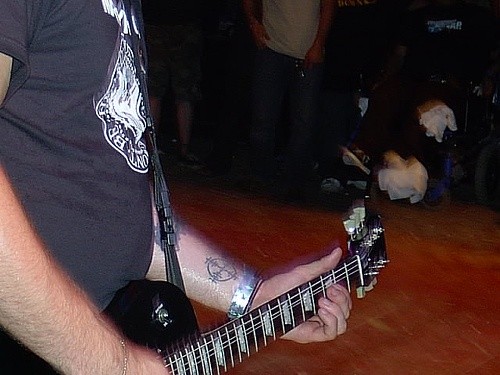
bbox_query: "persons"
[0,0,353,375]
[335,37,500,208]
[241,0,336,187]
[139,0,210,171]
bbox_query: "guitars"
[0,194,388,375]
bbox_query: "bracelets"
[226,268,265,321]
[118,334,129,375]
